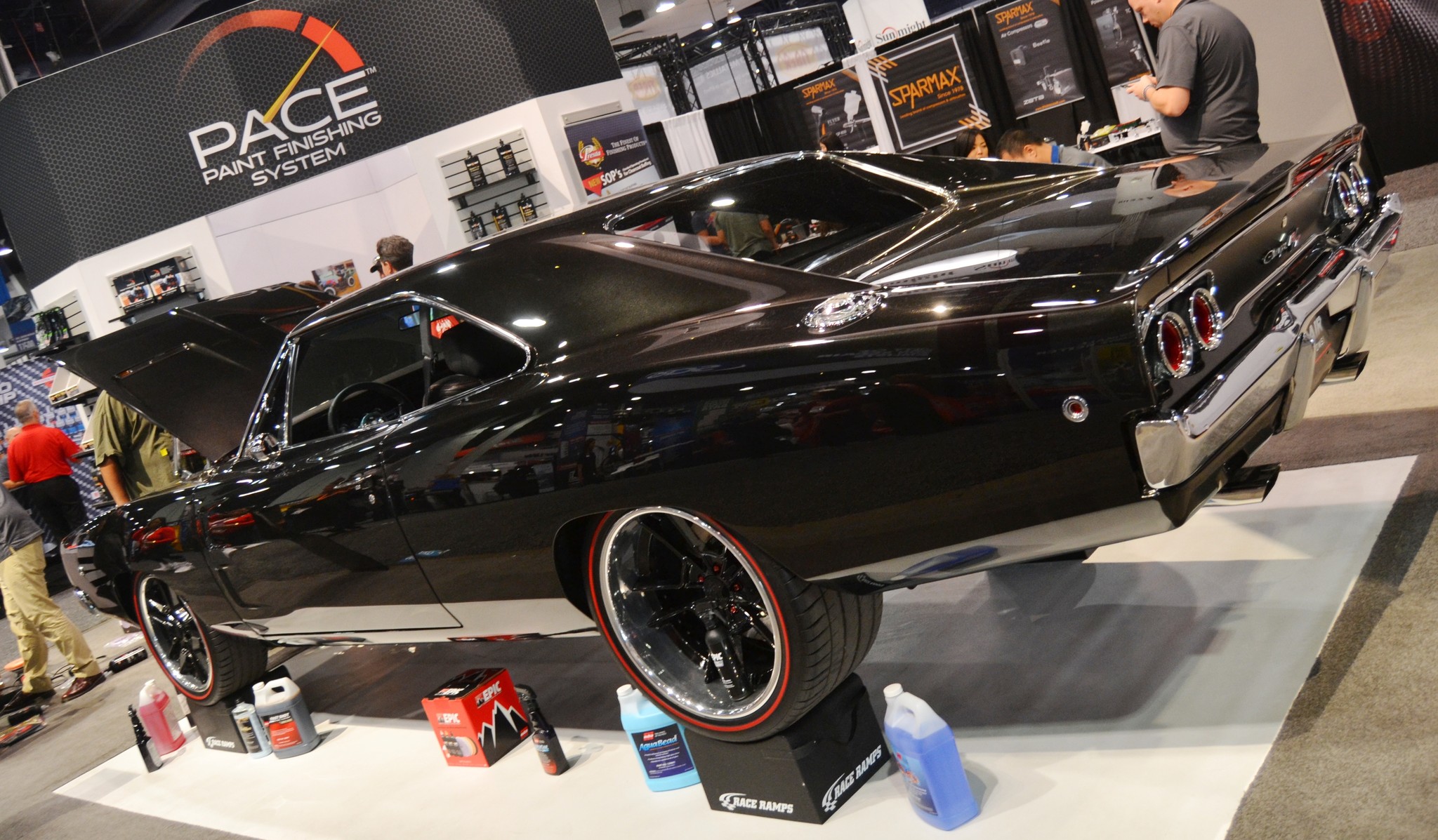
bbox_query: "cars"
[34,121,1402,745]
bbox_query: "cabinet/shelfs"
[435,126,550,245]
[107,284,210,327]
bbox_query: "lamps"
[725,0,743,25]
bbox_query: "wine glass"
[165,273,178,288]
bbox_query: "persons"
[369,259,384,281]
[691,210,730,256]
[0,425,24,490]
[1124,0,1261,157]
[90,389,179,506]
[953,127,990,159]
[130,517,176,561]
[577,437,599,485]
[377,236,414,276]
[0,483,107,713]
[93,509,129,579]
[716,212,779,261]
[8,399,89,543]
[819,132,843,151]
[995,127,1112,168]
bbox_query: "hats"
[369,255,386,273]
[375,234,415,264]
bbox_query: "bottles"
[175,685,195,728]
[88,465,114,502]
[492,202,512,232]
[517,193,538,223]
[8,704,50,727]
[617,684,700,790]
[883,682,980,830]
[524,693,570,775]
[232,698,273,758]
[441,730,463,757]
[129,711,163,772]
[252,677,321,759]
[134,285,146,300]
[463,150,488,189]
[467,211,488,241]
[106,646,148,673]
[496,138,520,178]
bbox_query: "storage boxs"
[186,665,304,755]
[685,671,890,827]
[420,666,535,770]
[112,254,197,316]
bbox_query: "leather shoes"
[61,672,108,703]
[3,685,57,714]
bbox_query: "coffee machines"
[139,679,184,756]
